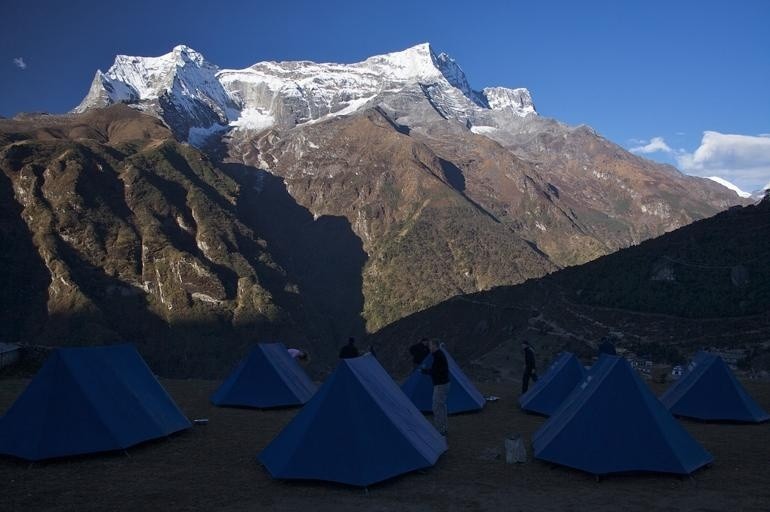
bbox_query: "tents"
[516,351,585,417]
[531,354,720,479]
[398,343,488,416]
[1,339,197,468]
[255,352,450,487]
[518,340,538,394]
[207,339,317,408]
[654,352,769,424]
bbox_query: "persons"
[410,337,428,367]
[337,335,358,359]
[287,344,308,361]
[421,339,451,437]
[370,346,376,356]
